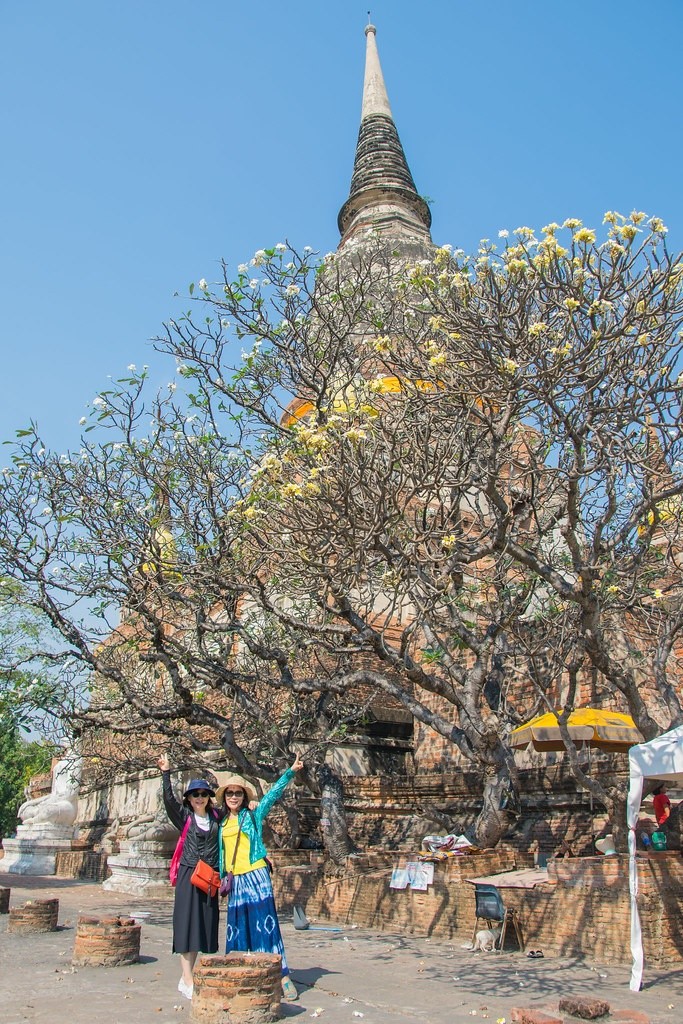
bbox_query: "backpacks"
[169,810,218,887]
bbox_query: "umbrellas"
[506,706,645,856]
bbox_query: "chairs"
[469,884,524,955]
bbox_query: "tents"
[627,725,683,991]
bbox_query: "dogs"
[470,925,502,952]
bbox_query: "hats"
[183,780,216,797]
[215,776,254,806]
[594,834,615,853]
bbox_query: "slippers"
[527,950,537,958]
[536,951,543,957]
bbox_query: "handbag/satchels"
[219,871,233,897]
[190,859,221,906]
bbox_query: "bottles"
[652,831,667,851]
[641,831,653,850]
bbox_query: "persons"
[17,737,83,824]
[120,770,186,841]
[157,752,259,999]
[652,784,671,828]
[215,752,304,1000]
[101,811,120,841]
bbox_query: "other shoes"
[282,978,297,1000]
[178,979,194,999]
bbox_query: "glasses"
[189,791,209,797]
[225,791,244,797]
